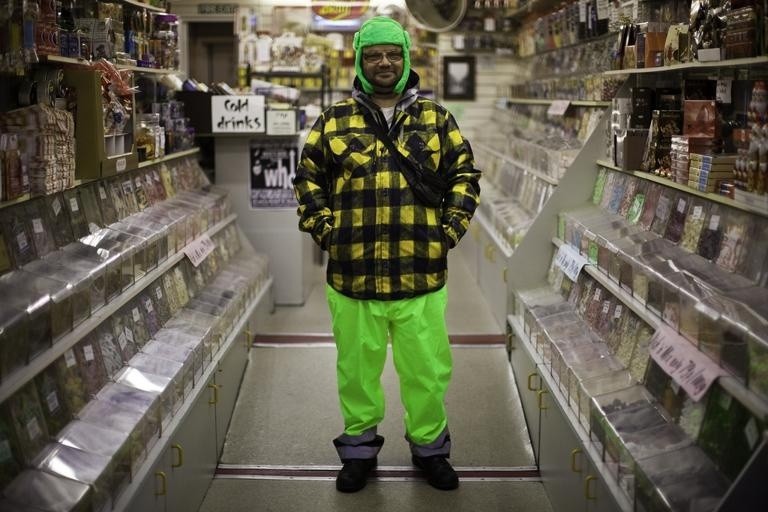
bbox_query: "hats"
[352,16,413,96]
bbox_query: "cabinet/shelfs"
[1,0,277,510]
[213,103,322,307]
[453,0,768,512]
[243,32,337,113]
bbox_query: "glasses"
[361,52,403,64]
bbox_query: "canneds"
[136,114,160,160]
[150,113,165,160]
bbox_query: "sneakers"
[412,453,460,491]
[335,454,377,494]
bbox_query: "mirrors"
[404,2,469,32]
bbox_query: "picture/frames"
[440,55,476,100]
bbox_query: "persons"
[291,17,484,494]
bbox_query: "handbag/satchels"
[395,152,448,207]
